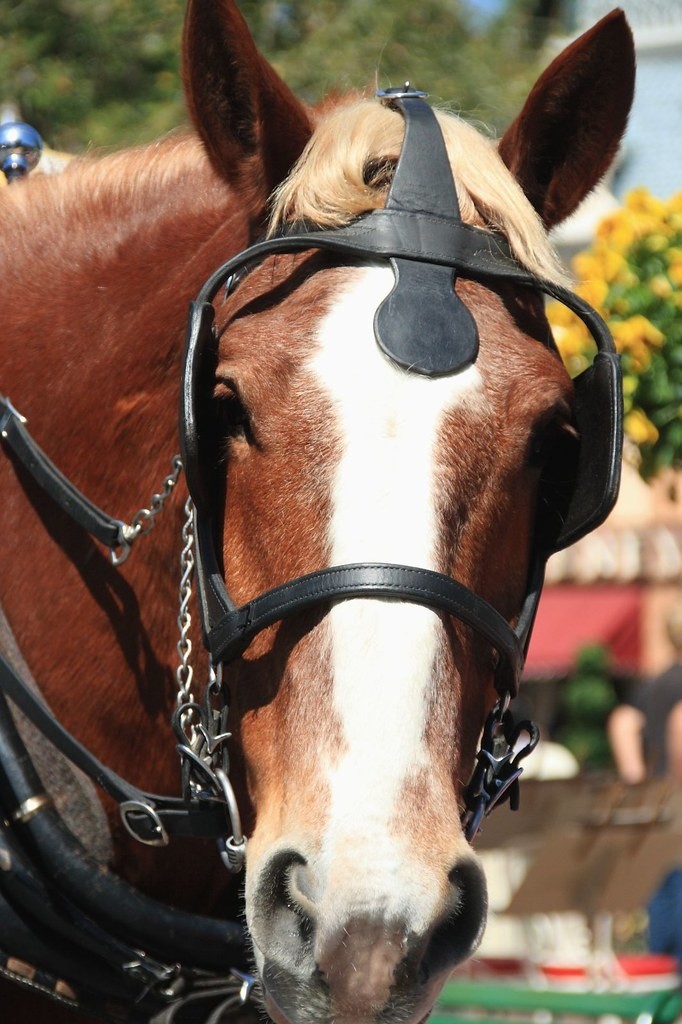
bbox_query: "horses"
[1,0,640,1024]
